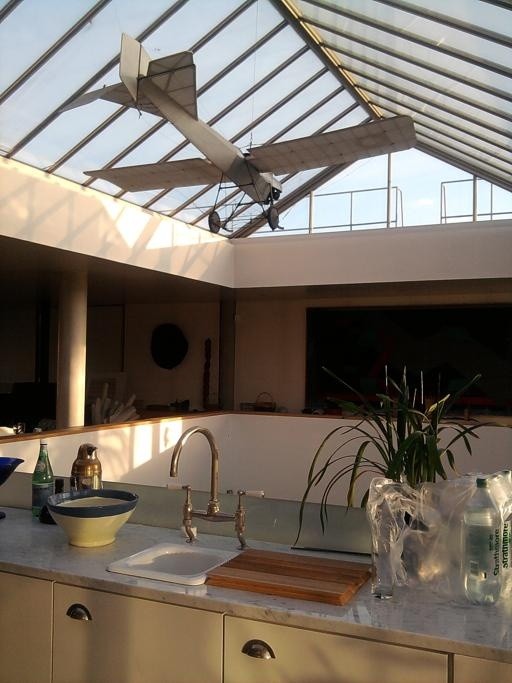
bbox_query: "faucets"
[170,425,221,513]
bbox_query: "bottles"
[31,439,54,517]
[457,470,512,604]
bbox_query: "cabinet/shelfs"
[452,653,512,683]
[223,615,450,683]
[0,571,54,683]
[51,582,224,683]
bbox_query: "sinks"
[108,541,236,586]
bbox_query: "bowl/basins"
[46,488,139,547]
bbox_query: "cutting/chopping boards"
[205,549,377,605]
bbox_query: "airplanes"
[55,32,421,235]
